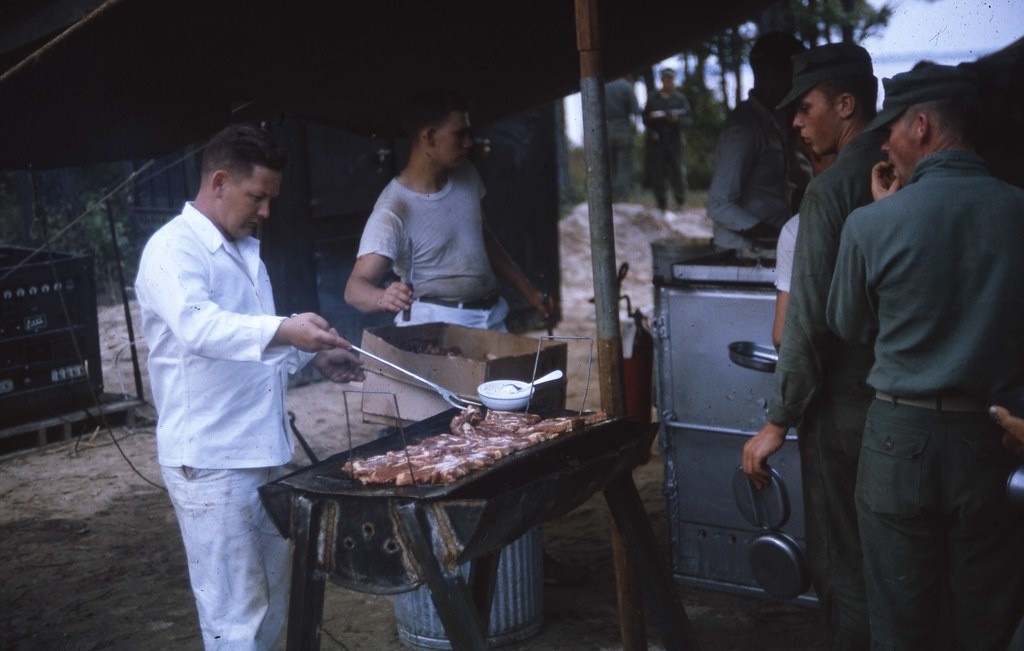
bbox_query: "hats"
[662,69,676,77]
[776,44,872,110]
[862,61,976,134]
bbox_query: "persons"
[344,91,556,332]
[826,59,1024,651]
[706,31,817,268]
[743,41,902,651]
[643,69,694,211]
[134,124,365,651]
[605,77,643,204]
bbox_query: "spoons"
[499,370,563,393]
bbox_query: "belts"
[418,294,500,309]
[874,386,995,414]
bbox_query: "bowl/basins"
[478,380,535,410]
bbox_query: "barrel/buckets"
[394,523,544,651]
[649,239,716,319]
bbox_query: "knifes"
[402,238,413,321]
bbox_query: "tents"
[0,0,768,651]
